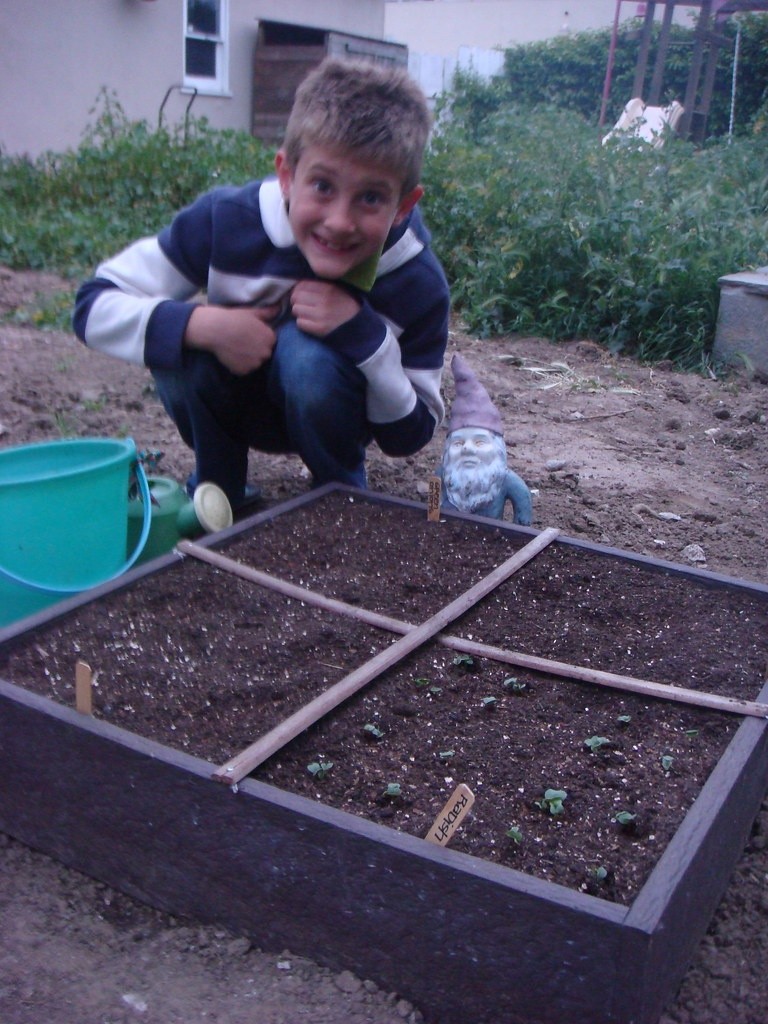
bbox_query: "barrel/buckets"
[0,437,152,629]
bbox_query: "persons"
[438,352,535,526]
[71,54,451,520]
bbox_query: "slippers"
[181,483,260,510]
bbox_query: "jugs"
[124,474,233,566]
